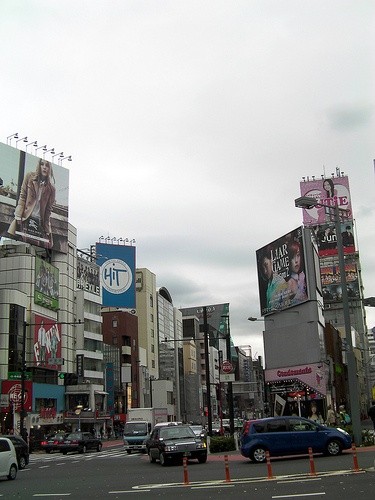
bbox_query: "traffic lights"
[23,428,28,438]
[60,372,77,380]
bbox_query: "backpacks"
[345,413,351,424]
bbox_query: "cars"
[0,438,18,480]
[1,435,29,469]
[41,434,67,453]
[61,431,103,455]
[240,416,353,463]
[155,419,246,440]
[145,425,207,466]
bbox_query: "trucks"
[122,407,168,454]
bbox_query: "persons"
[326,405,336,428]
[36,261,55,298]
[38,320,47,366]
[100,425,111,440]
[282,241,307,303]
[78,262,100,296]
[339,405,350,432]
[7,160,55,248]
[49,328,58,368]
[329,226,337,248]
[341,226,354,247]
[320,227,330,249]
[50,424,72,437]
[75,427,96,439]
[113,425,124,440]
[8,428,16,436]
[22,427,44,455]
[256,246,287,313]
[369,401,375,430]
[308,402,321,424]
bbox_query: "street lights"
[295,196,364,444]
[221,314,235,438]
